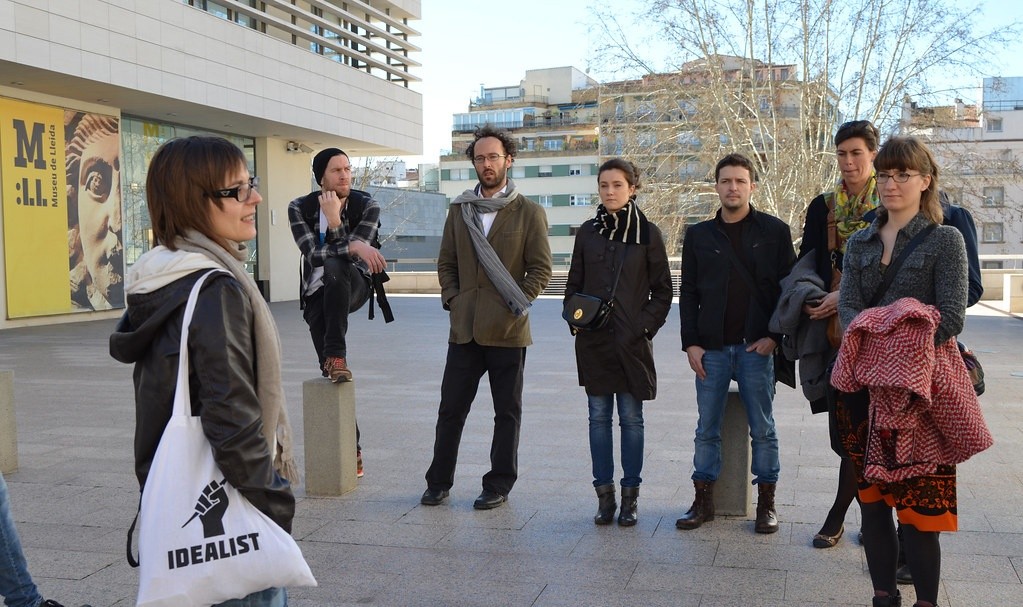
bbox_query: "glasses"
[872,170,920,184]
[840,121,876,137]
[204,176,259,203]
[472,155,506,163]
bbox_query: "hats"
[312,148,347,185]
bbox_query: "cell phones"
[805,299,823,308]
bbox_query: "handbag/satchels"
[136,268,317,607]
[961,350,983,396]
[564,291,611,331]
[828,268,845,348]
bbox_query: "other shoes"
[814,524,844,548]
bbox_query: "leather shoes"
[895,562,914,584]
[473,488,509,508]
[421,489,450,506]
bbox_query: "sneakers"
[355,452,364,476]
[325,356,353,383]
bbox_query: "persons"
[0,472,45,607]
[675,154,798,532]
[110,136,296,607]
[562,158,674,525]
[66,113,126,310]
[837,134,984,607]
[420,124,552,510]
[799,120,881,550]
[288,148,388,476]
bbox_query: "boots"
[593,483,616,524]
[619,486,642,523]
[676,479,715,529]
[756,481,780,533]
